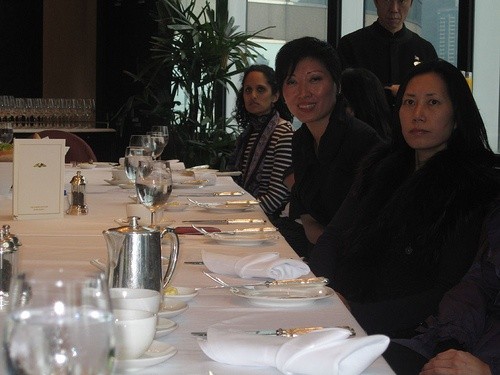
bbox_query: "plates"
[104,179,127,185]
[161,202,190,211]
[173,179,209,189]
[118,184,130,189]
[203,205,247,213]
[76,162,118,167]
[155,287,199,337]
[114,219,131,226]
[205,235,279,245]
[113,340,177,371]
[229,287,334,307]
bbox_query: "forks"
[192,224,279,236]
[203,271,331,289]
[187,198,261,208]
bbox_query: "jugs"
[90,216,179,294]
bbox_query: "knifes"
[179,192,244,196]
[192,326,355,337]
[182,218,266,224]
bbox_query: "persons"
[274,36,500,375]
[233,65,296,220]
[336,0,437,98]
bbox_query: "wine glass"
[125,125,173,228]
[0,95,97,128]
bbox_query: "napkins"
[198,323,390,374]
[202,252,310,280]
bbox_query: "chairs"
[30,130,97,163]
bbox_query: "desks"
[12,128,116,161]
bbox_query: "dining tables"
[0,159,395,375]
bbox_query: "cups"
[461,70,472,93]
[0,122,14,144]
[113,309,157,360]
[112,169,124,179]
[109,288,162,314]
[4,266,112,375]
[126,203,155,225]
[119,158,125,166]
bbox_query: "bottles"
[0,224,21,291]
[70,172,88,214]
